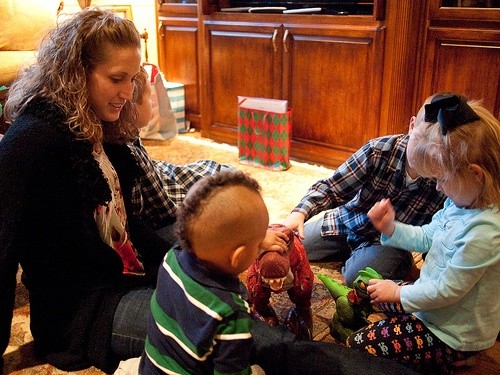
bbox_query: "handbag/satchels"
[136,61,178,142]
[236,95,294,172]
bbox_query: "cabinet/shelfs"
[155,3,201,132]
[417,8,500,121]
[198,12,382,164]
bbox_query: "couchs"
[0,0,83,102]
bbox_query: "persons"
[0,8,395,375]
[278,88,448,289]
[345,97,499,375]
[137,168,290,375]
